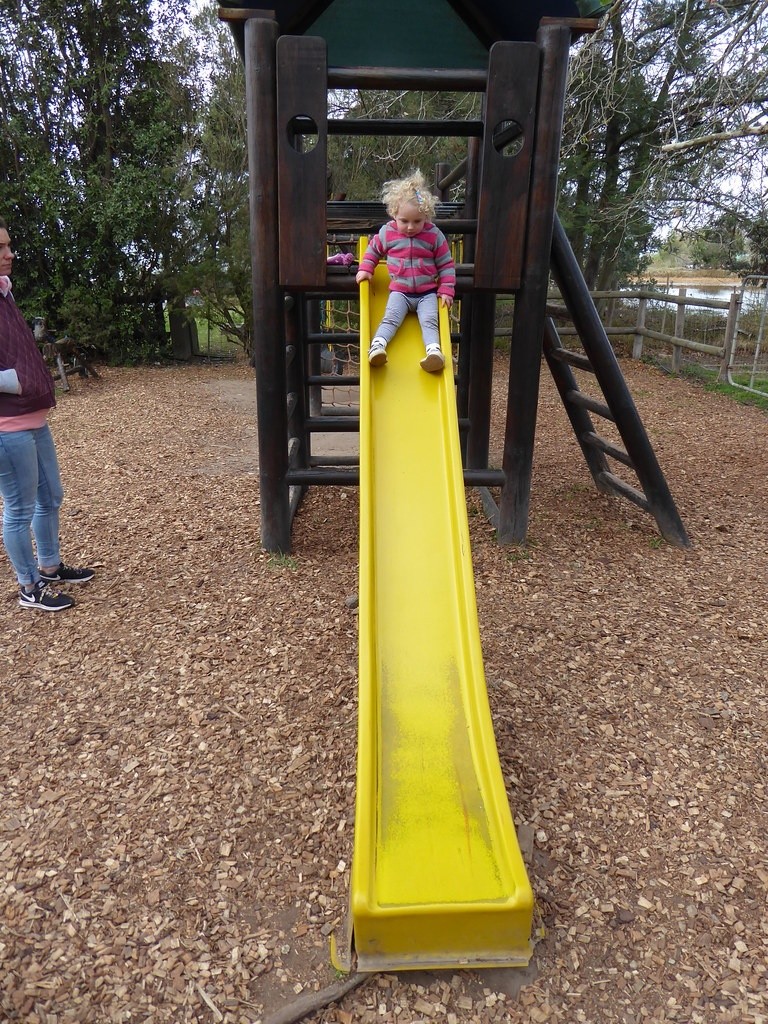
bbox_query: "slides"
[345,234,539,975]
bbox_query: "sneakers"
[37,562,95,584]
[19,584,75,612]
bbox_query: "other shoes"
[419,349,445,373]
[368,342,388,368]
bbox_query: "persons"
[354,169,460,374]
[0,212,95,611]
